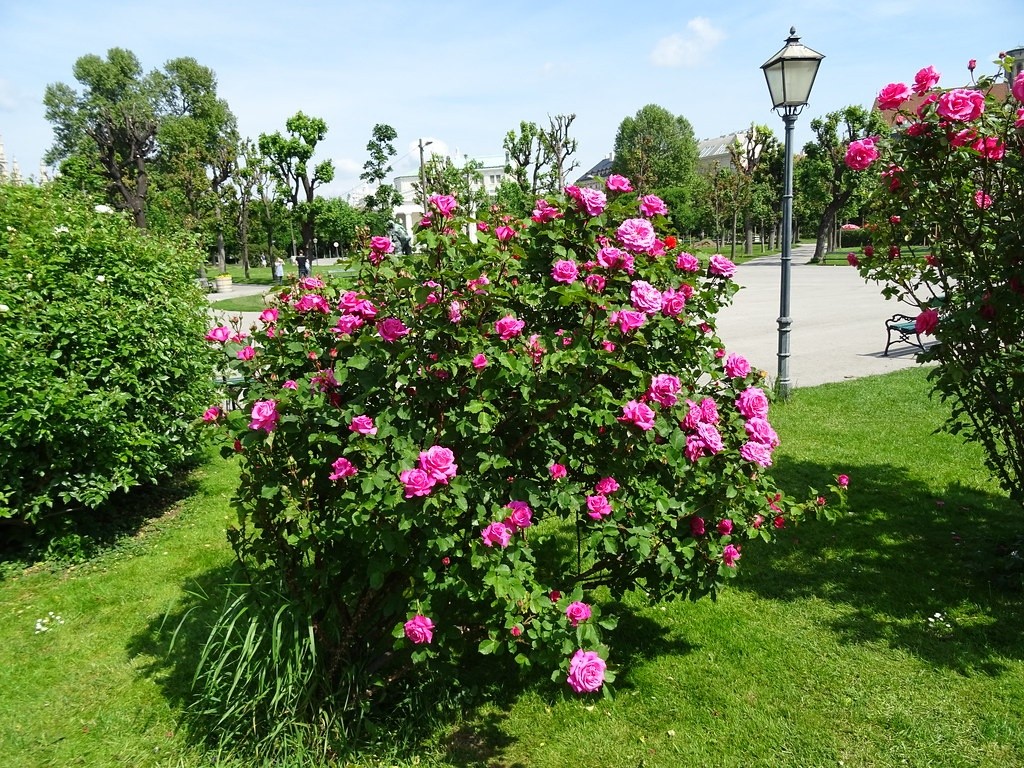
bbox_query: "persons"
[261,252,266,268]
[274,257,285,286]
[295,250,309,282]
[387,220,413,256]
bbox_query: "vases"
[216,279,232,292]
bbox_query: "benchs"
[883,314,928,356]
[195,278,214,293]
[328,268,357,281]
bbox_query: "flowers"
[214,272,232,279]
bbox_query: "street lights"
[759,26,827,401]
[313,237,320,268]
[419,139,435,217]
